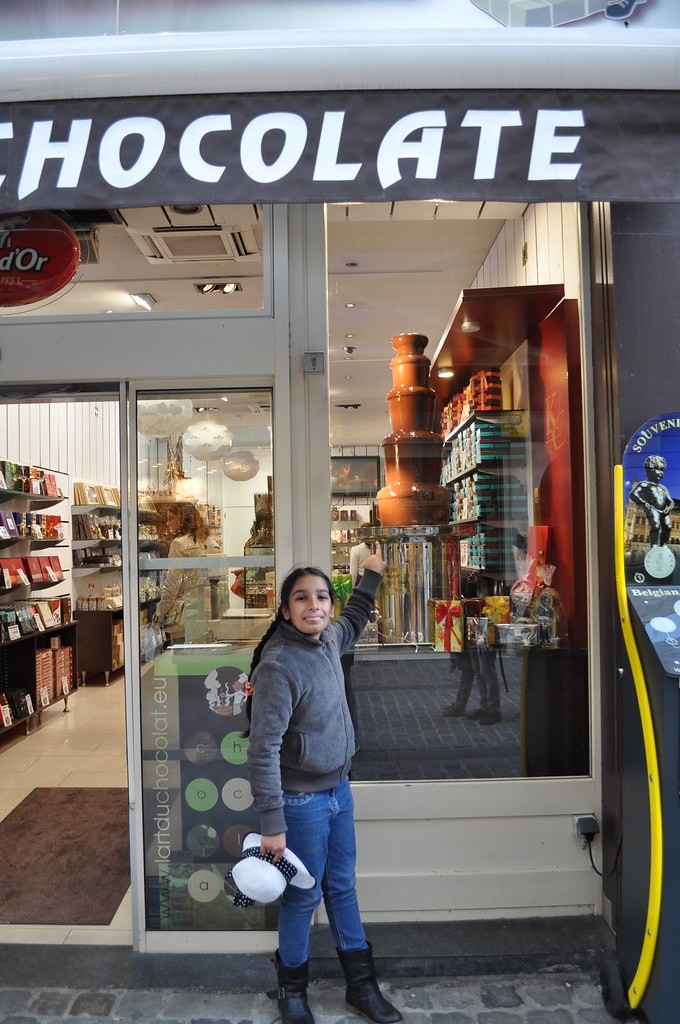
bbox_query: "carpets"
[0,787,181,926]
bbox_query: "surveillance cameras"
[344,347,356,354]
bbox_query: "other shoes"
[441,703,466,717]
[478,711,501,725]
[468,706,487,719]
[448,663,456,672]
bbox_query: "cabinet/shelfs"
[71,502,158,579]
[72,596,171,686]
[0,457,79,736]
[442,408,526,580]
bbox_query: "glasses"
[166,516,176,524]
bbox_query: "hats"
[225,833,316,908]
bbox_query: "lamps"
[127,399,193,438]
[460,321,480,332]
[222,449,259,481]
[221,283,236,295]
[199,284,216,295]
[437,368,456,378]
[182,399,232,461]
[130,294,154,311]
[196,408,204,412]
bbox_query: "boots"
[336,939,404,1024]
[270,945,315,1024]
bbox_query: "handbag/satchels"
[142,626,167,662]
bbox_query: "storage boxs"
[427,368,526,653]
[1,462,73,727]
[112,609,149,665]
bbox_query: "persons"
[444,545,501,725]
[244,506,275,611]
[346,523,377,623]
[152,503,222,645]
[245,539,404,1024]
